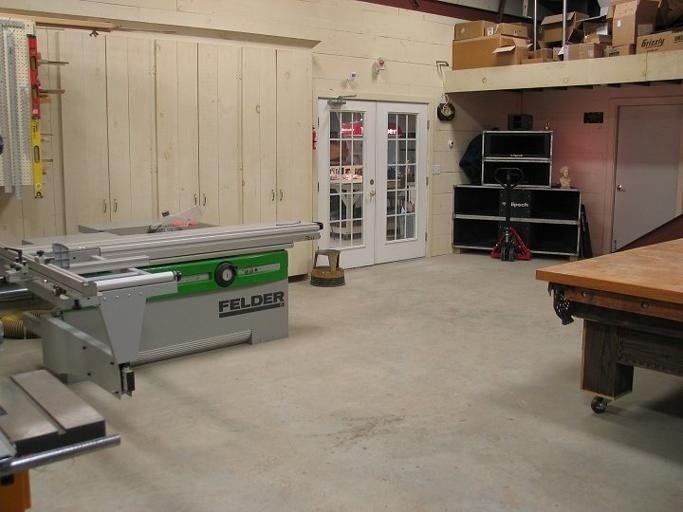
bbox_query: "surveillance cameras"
[346,71,357,81]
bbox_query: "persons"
[558,166,572,189]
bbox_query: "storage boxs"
[452,1,683,69]
